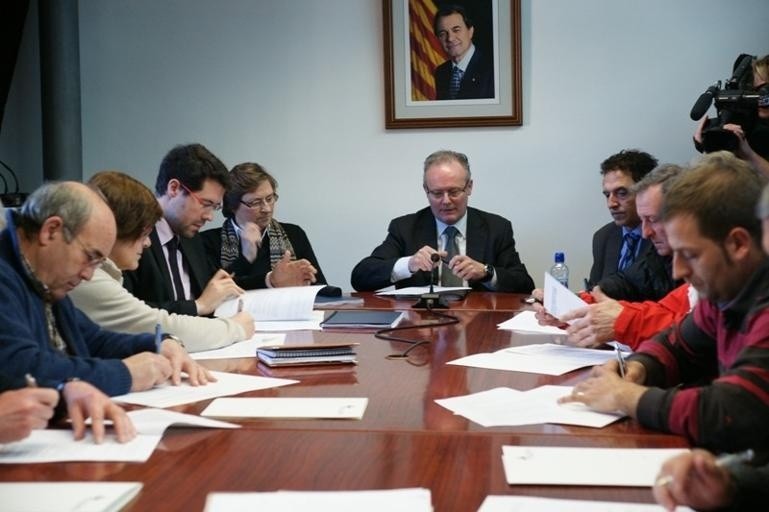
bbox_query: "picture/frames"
[380,0,524,133]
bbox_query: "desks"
[0,287,720,511]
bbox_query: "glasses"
[65,223,105,271]
[240,193,279,208]
[426,180,469,197]
[180,183,222,211]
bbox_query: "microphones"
[411,254,450,308]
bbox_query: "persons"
[351,151,536,292]
[531,150,769,352]
[430,4,494,99]
[690,52,768,165]
[555,147,769,446]
[198,162,329,286]
[0,180,218,445]
[652,183,769,511]
[66,170,255,352]
[122,142,317,318]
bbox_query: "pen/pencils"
[24,373,37,387]
[614,342,626,377]
[441,257,466,277]
[657,448,755,488]
[238,299,243,312]
[155,324,161,353]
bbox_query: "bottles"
[550,252,570,289]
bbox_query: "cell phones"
[520,295,535,303]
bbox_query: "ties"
[618,233,638,270]
[165,235,186,303]
[440,226,464,288]
[448,66,461,99]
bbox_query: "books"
[319,311,404,328]
[256,343,358,368]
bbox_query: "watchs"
[479,264,494,282]
